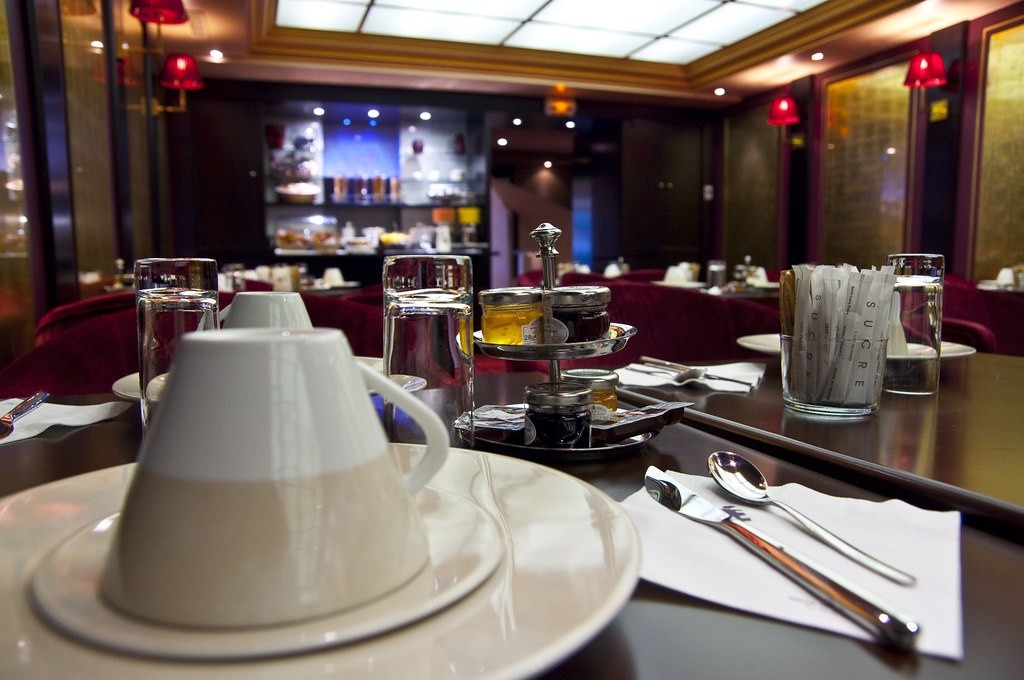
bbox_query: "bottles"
[562,368,618,415]
[552,286,610,344]
[479,287,544,346]
[734,265,748,293]
[525,383,592,449]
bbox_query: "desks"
[2,392,1023,678]
[614,353,1024,522]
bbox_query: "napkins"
[0,397,131,444]
[617,363,767,391]
[611,468,964,658]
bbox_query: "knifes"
[0,391,51,440]
[644,464,919,649]
[641,355,751,386]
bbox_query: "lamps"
[905,52,959,112]
[767,89,808,149]
[124,1,189,56]
[153,52,203,114]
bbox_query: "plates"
[1,441,639,675]
[34,489,505,660]
[111,356,386,400]
[737,333,977,360]
[652,281,705,289]
[755,283,781,288]
[146,373,427,402]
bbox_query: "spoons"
[627,364,705,383]
[709,451,915,586]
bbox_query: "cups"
[324,171,401,201]
[778,335,891,421]
[882,253,945,395]
[222,264,301,293]
[136,259,219,438]
[97,288,444,630]
[382,255,475,450]
[707,260,728,289]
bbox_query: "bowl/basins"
[275,185,320,202]
